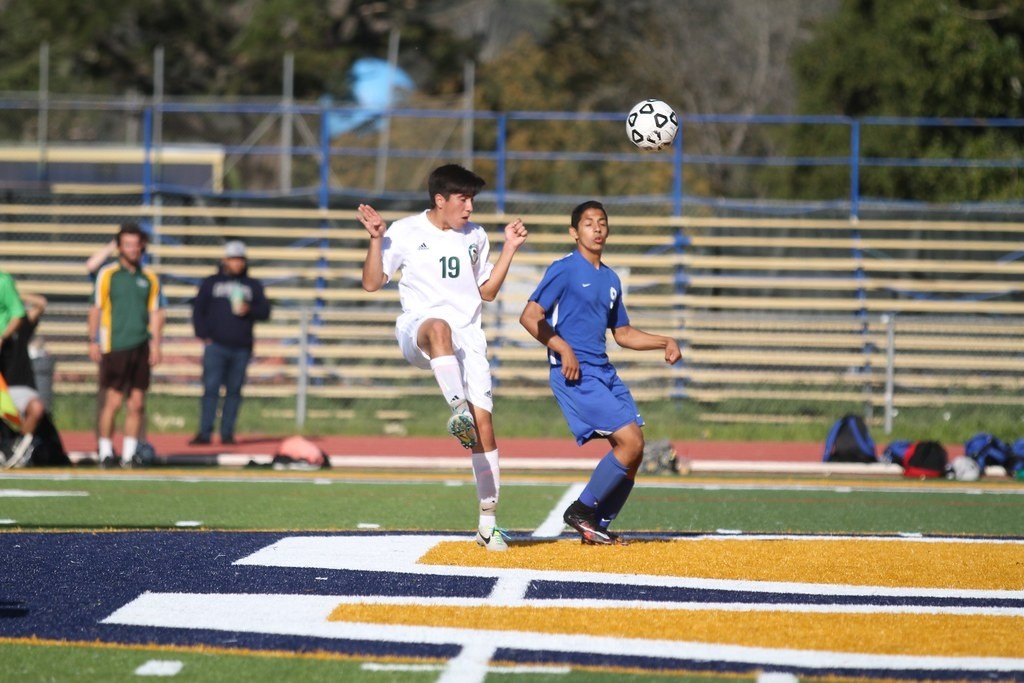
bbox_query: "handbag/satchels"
[886,435,1024,481]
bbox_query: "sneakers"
[476,526,511,551]
[581,534,627,545]
[563,503,612,544]
[449,412,478,449]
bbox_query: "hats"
[224,242,245,258]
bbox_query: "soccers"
[952,455,980,482]
[626,99,679,152]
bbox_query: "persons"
[188,241,271,446]
[520,201,681,546]
[0,272,71,466]
[85,223,167,469]
[355,163,528,550]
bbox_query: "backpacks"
[824,412,876,461]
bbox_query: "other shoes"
[224,439,236,445]
[190,436,210,446]
